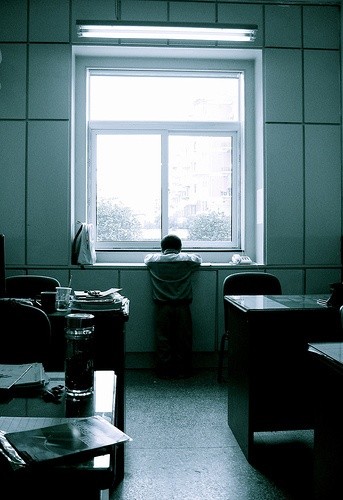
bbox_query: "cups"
[56,287,72,311]
[35,292,57,312]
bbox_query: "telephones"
[231,253,253,265]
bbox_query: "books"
[0,363,49,389]
[0,415,129,473]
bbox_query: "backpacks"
[72,220,97,265]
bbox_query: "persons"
[146,236,200,379]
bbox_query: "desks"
[0,297,130,500]
[223,293,343,490]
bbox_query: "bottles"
[65,314,95,396]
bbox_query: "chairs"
[5,276,61,300]
[216,273,282,383]
[1,299,52,367]
